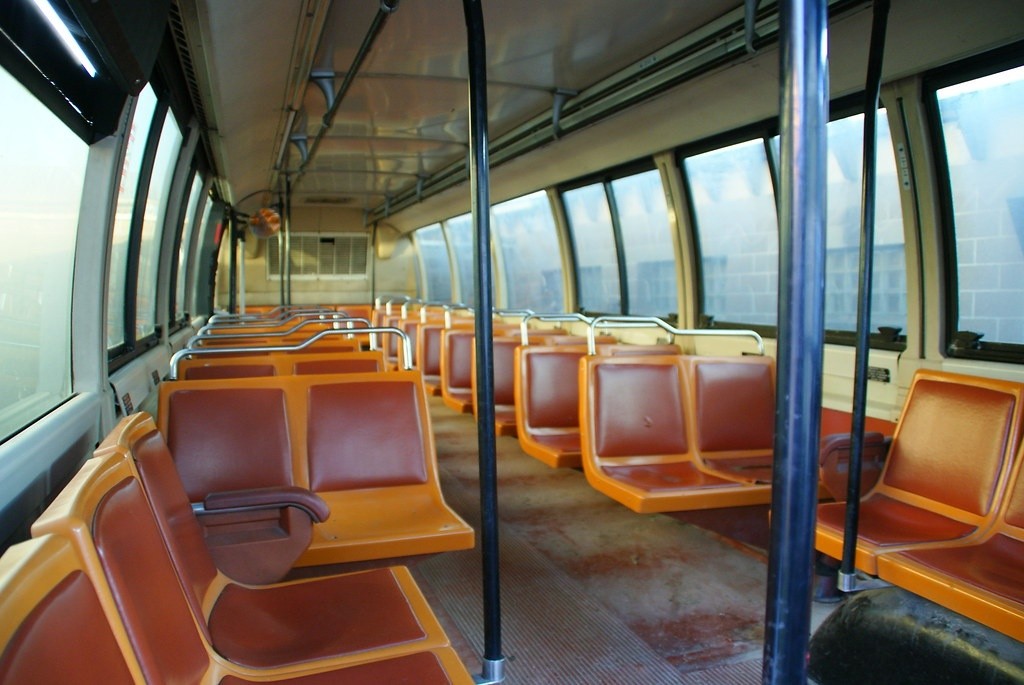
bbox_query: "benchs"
[0,293,1024,685]
